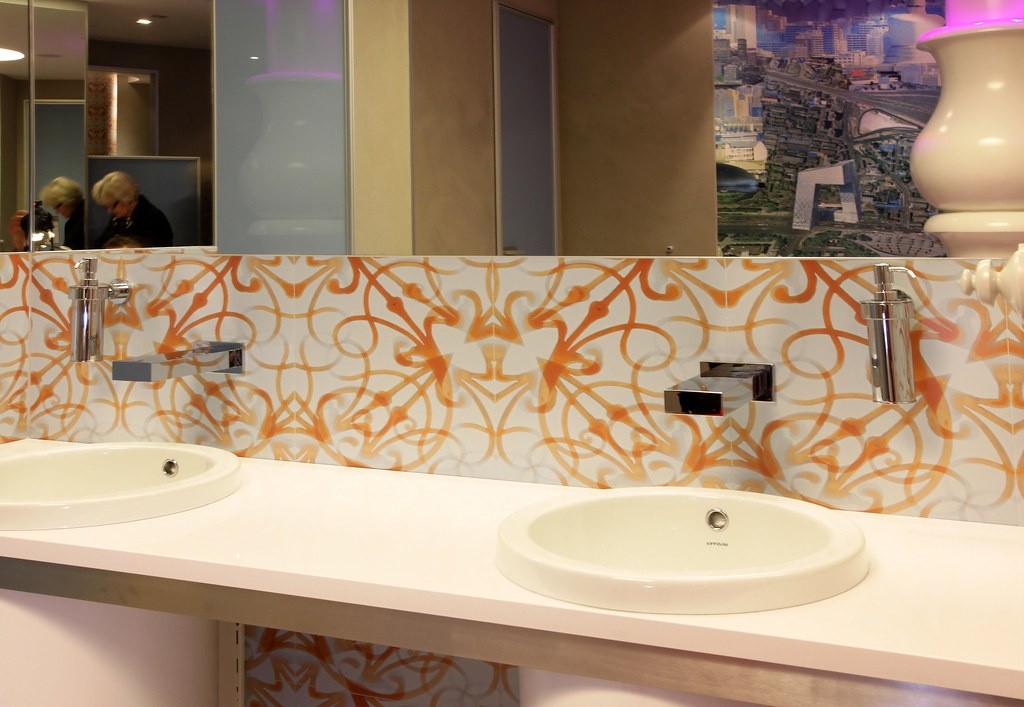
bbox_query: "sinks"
[0,442,241,530]
[497,487,870,615]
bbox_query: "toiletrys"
[860,262,919,403]
[67,256,109,364]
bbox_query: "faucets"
[111,341,246,382]
[663,361,775,416]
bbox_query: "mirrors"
[0,0,1024,258]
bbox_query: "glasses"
[54,204,62,210]
[105,200,119,210]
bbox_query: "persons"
[93,169,175,247]
[38,177,85,250]
